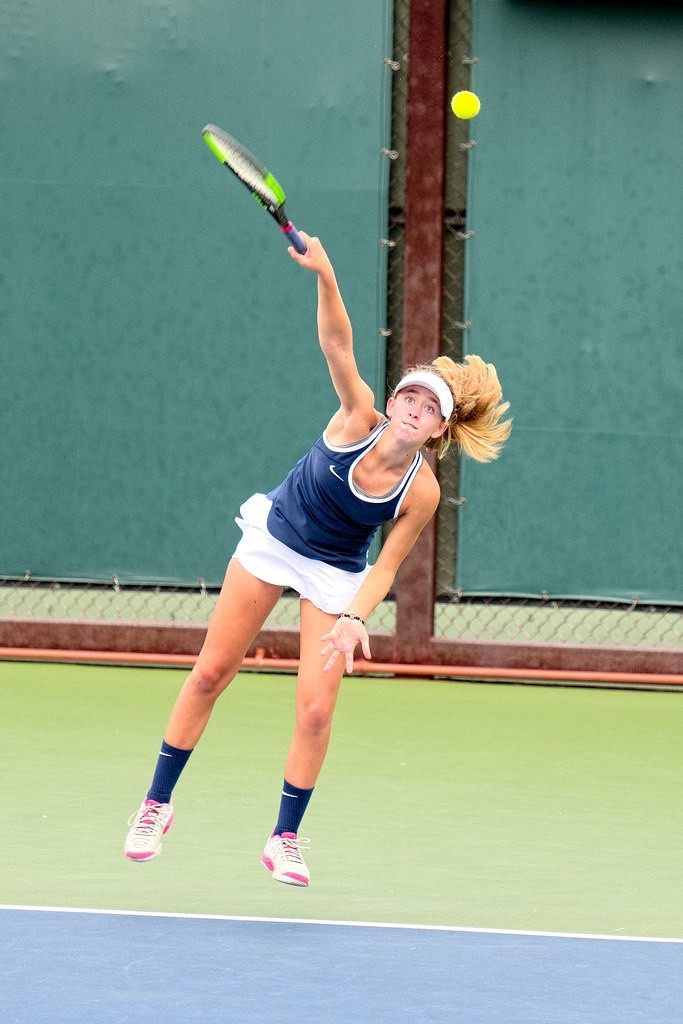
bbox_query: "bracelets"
[337,614,365,625]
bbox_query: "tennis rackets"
[201,124,307,255]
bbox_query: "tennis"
[450,89,482,121]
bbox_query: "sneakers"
[259,828,309,887]
[124,796,176,862]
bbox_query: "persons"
[123,228,514,888]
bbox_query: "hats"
[393,371,453,422]
